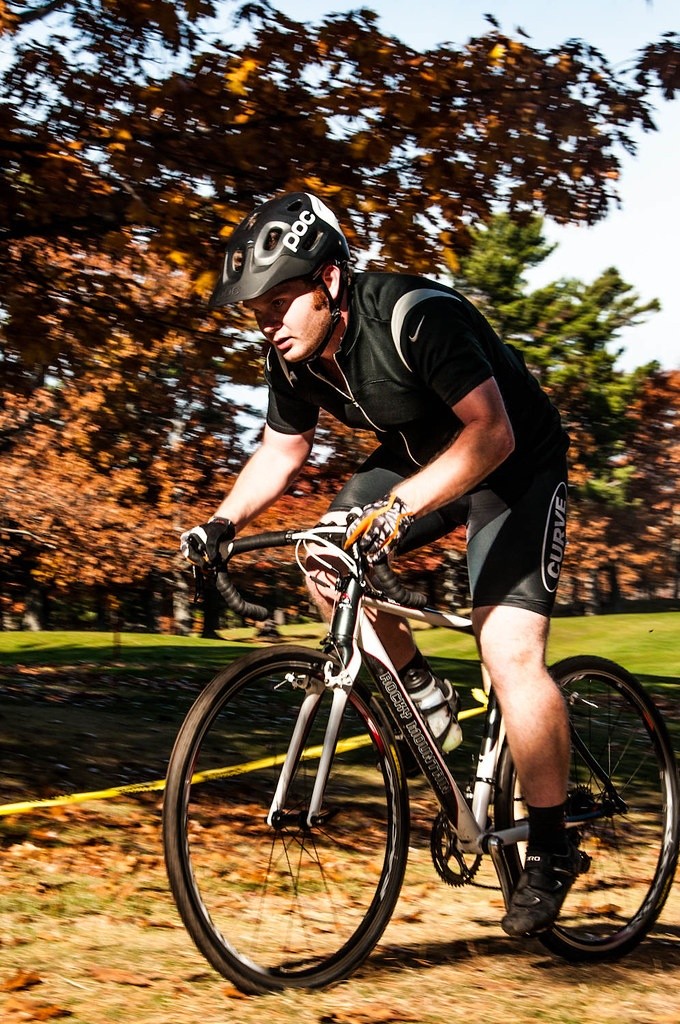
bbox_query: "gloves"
[180,515,235,574]
[344,491,416,565]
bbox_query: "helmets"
[208,192,351,306]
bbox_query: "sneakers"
[376,674,457,778]
[500,827,582,940]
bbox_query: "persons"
[178,191,592,936]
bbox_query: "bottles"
[403,668,463,754]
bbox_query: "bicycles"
[159,505,680,996]
